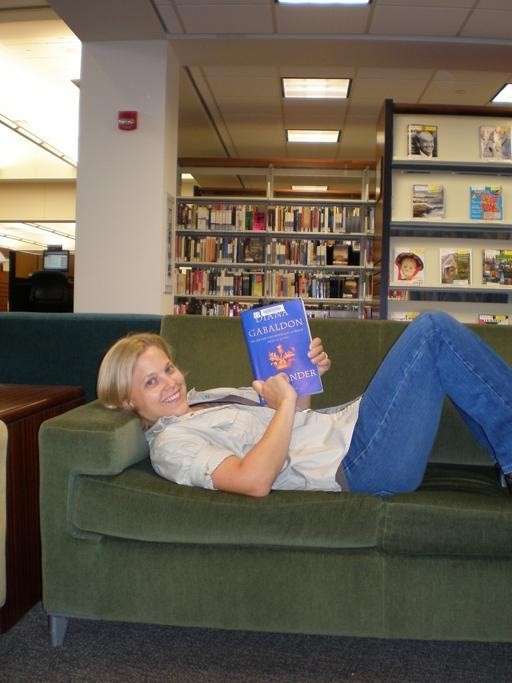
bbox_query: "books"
[407,123,440,161]
[479,126,511,161]
[175,201,373,318]
[481,248,512,289]
[478,313,510,325]
[470,183,504,223]
[410,183,446,221]
[439,248,472,288]
[392,246,426,286]
[240,296,324,403]
[387,310,420,321]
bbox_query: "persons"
[97,309,511,499]
[413,131,437,156]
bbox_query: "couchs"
[37,317,511,647]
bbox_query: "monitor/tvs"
[42,250,69,273]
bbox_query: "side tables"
[0,383,88,633]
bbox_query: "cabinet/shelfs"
[173,194,383,319]
[371,99,512,320]
